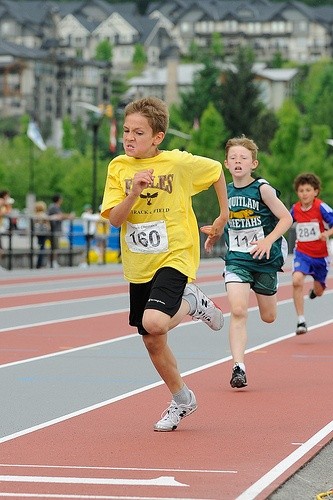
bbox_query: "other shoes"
[309,288,317,300]
[296,321,307,335]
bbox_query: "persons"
[49,194,76,268]
[205,137,293,389]
[34,200,68,269]
[99,97,229,432]
[0,190,15,231]
[288,173,333,334]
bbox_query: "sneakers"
[153,390,198,431]
[230,361,246,389]
[182,282,223,331]
